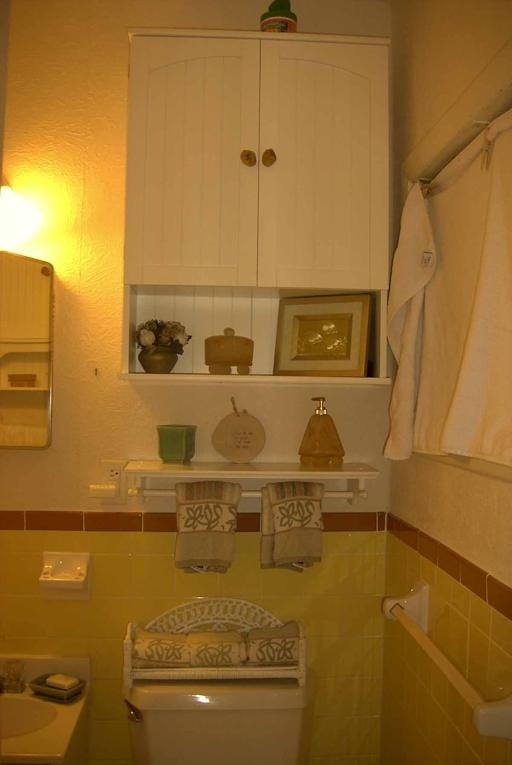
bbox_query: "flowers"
[133,319,191,355]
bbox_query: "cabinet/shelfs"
[114,26,394,387]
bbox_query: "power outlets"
[97,458,127,506]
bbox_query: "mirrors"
[0,251,55,451]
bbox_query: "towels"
[382,126,512,467]
[259,481,325,574]
[132,621,300,669]
[173,480,243,576]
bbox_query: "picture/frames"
[272,293,373,378]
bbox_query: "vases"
[137,345,177,373]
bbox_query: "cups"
[154,420,199,466]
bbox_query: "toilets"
[125,679,307,765]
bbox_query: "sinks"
[0,684,90,764]
